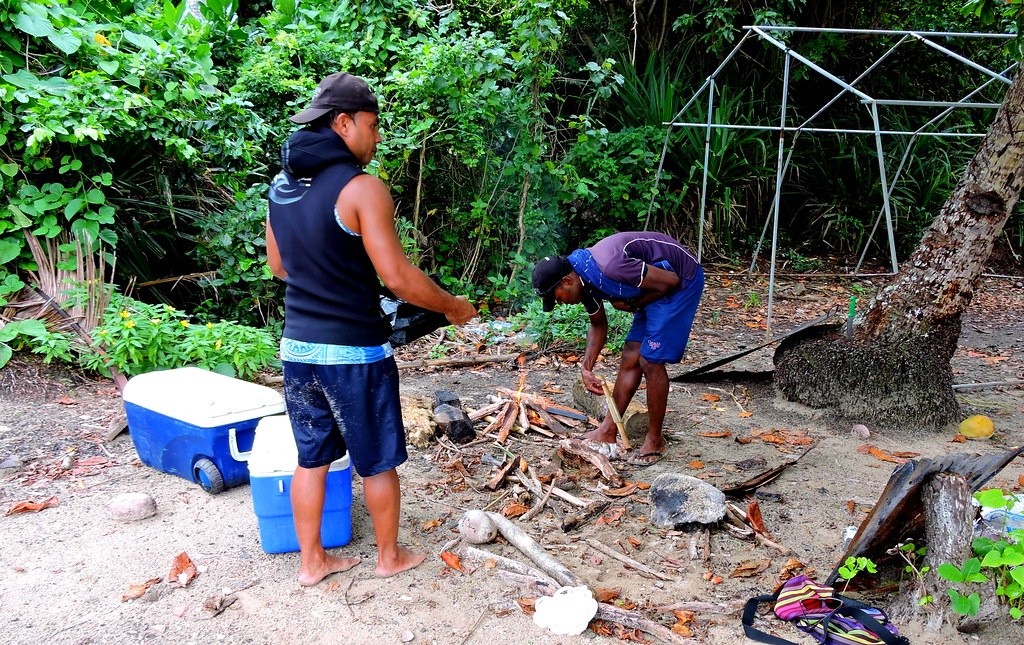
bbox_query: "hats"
[288,72,379,124]
[532,256,571,312]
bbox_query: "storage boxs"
[122,366,288,496]
[246,413,353,554]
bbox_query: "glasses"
[799,598,844,629]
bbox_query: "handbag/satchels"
[742,576,910,645]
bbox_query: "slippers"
[630,446,669,466]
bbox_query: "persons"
[532,231,704,465]
[267,72,478,586]
